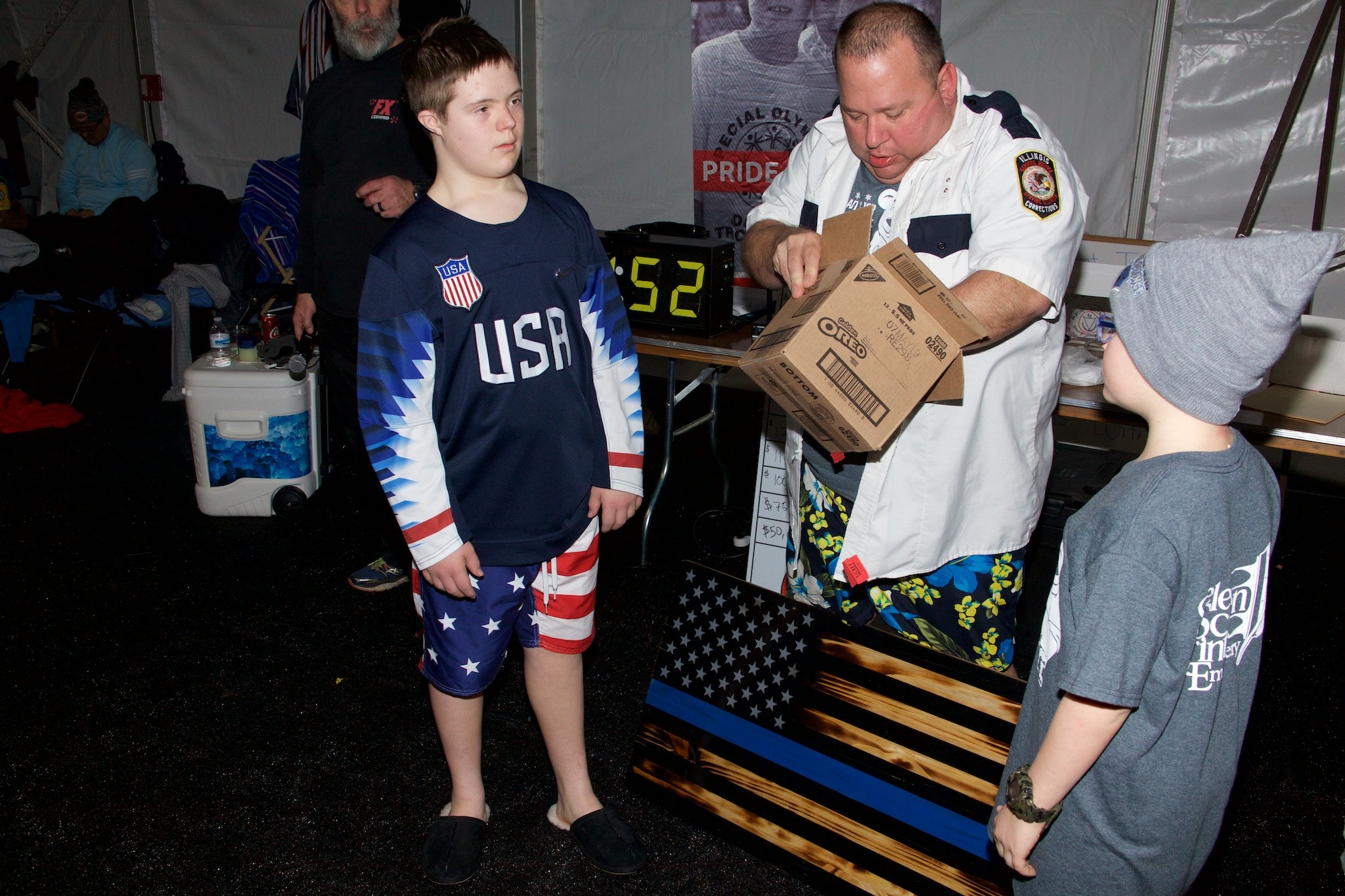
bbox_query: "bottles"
[209,317,231,367]
[237,333,259,362]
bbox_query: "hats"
[1111,231,1345,425]
[67,77,108,125]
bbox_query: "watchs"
[1005,764,1063,834]
[413,184,420,200]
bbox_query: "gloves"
[256,334,296,365]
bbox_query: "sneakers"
[348,556,412,591]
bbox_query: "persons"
[356,15,646,884]
[742,2,1092,676]
[292,0,411,592]
[691,0,839,241]
[797,0,874,78]
[983,231,1340,896]
[56,76,158,218]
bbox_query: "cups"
[224,325,252,361]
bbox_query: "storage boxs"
[737,207,989,460]
[184,351,327,518]
[1065,261,1128,339]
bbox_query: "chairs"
[0,184,236,409]
[240,154,304,316]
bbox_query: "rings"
[378,203,384,211]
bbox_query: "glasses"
[69,119,104,136]
[1096,314,1117,344]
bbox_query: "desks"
[631,314,1345,568]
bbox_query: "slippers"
[547,804,647,875]
[422,801,490,884]
